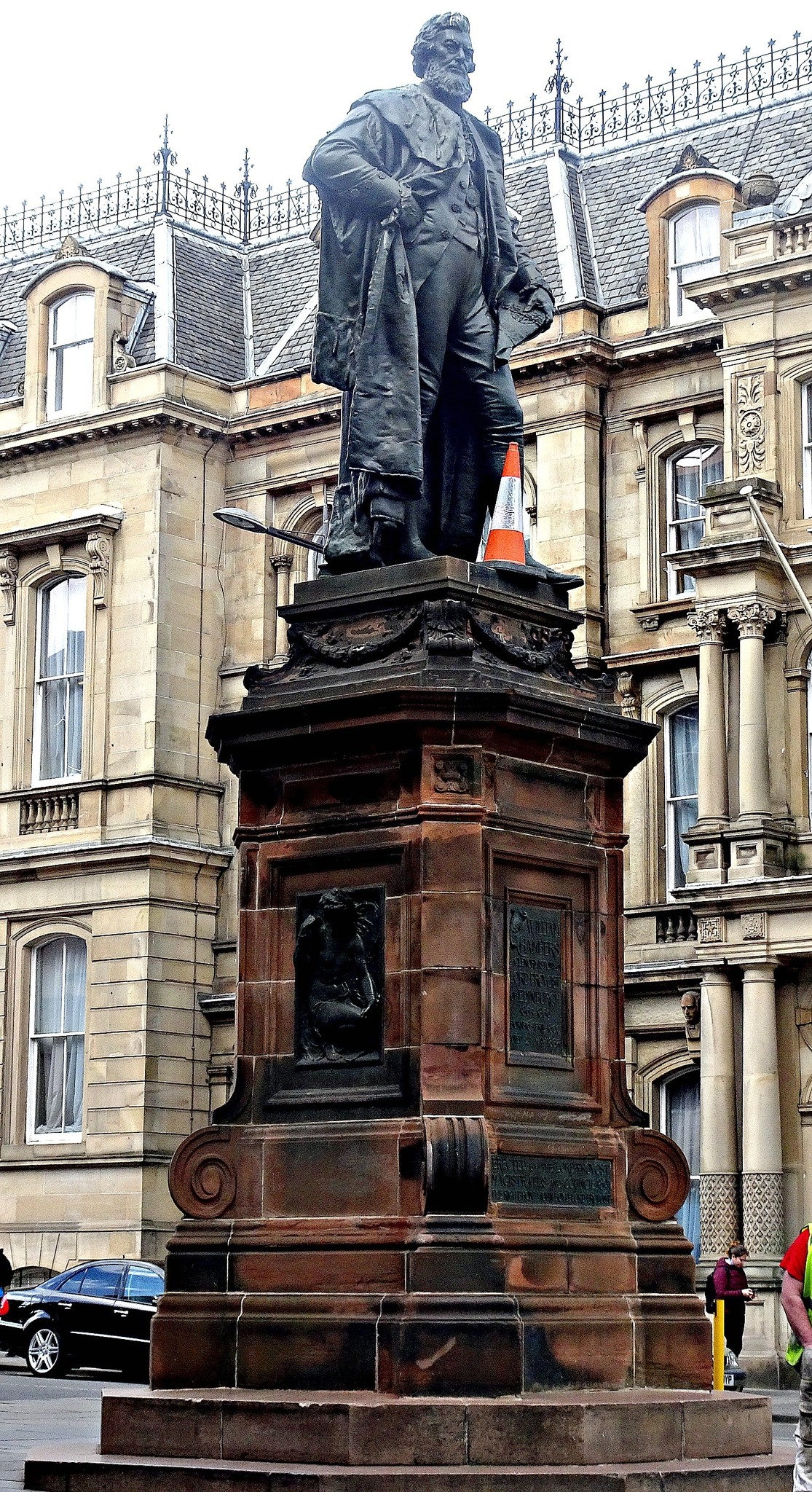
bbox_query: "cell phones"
[751,1288,757,1294]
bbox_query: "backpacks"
[0,1248,13,1287]
[705,1267,730,1314]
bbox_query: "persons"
[711,1241,753,1366]
[292,888,382,1059]
[779,1223,812,1492]
[302,11,585,590]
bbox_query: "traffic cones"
[476,442,547,581]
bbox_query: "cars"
[1,1260,165,1378]
